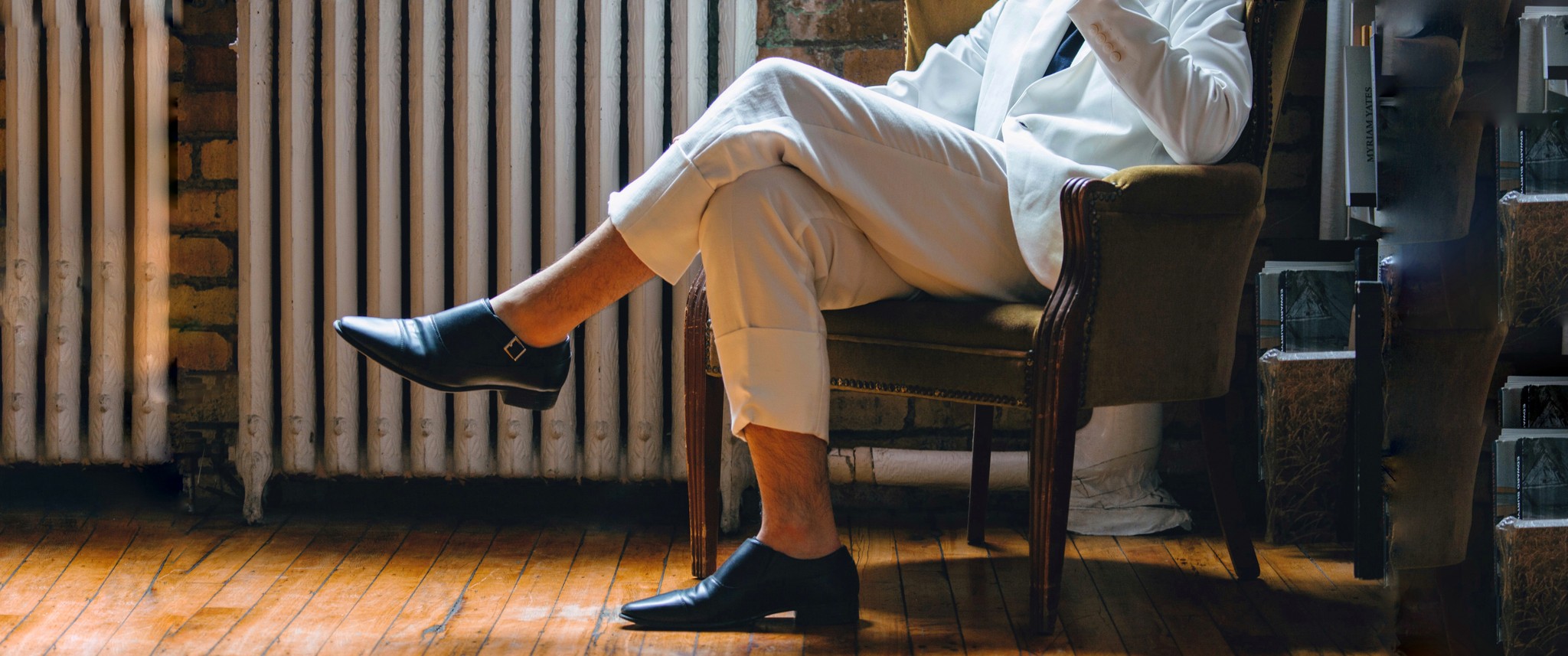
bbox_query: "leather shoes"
[619,536,860,626]
[333,297,572,411]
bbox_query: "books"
[1260,5,1568,656]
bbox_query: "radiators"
[0,0,172,466]
[229,0,760,537]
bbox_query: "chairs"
[685,0,1316,638]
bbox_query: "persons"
[333,0,1254,625]
